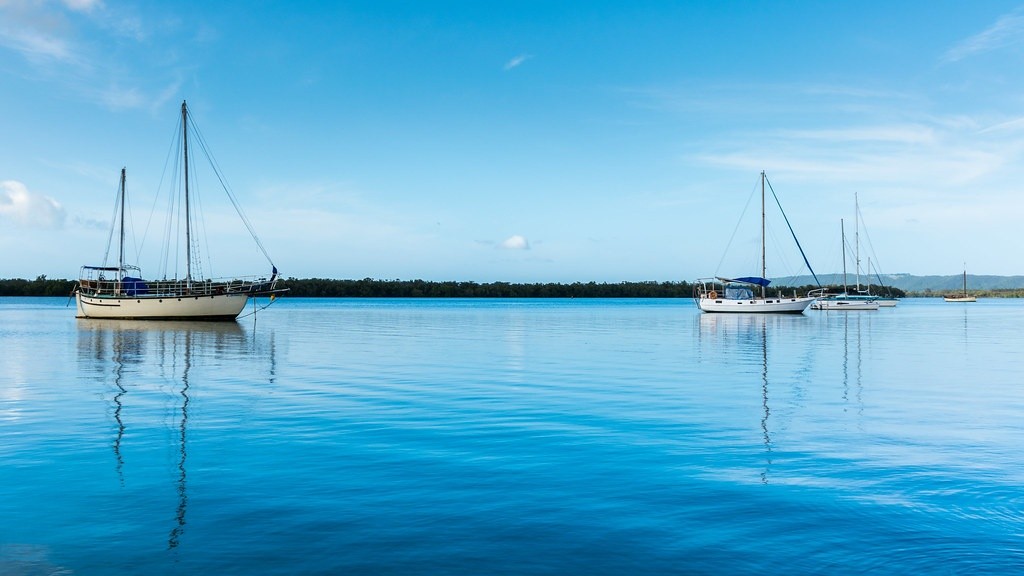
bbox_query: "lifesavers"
[710,291,717,299]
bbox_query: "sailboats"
[694,169,829,312]
[66,97,291,320]
[809,191,898,309]
[944,259,978,302]
